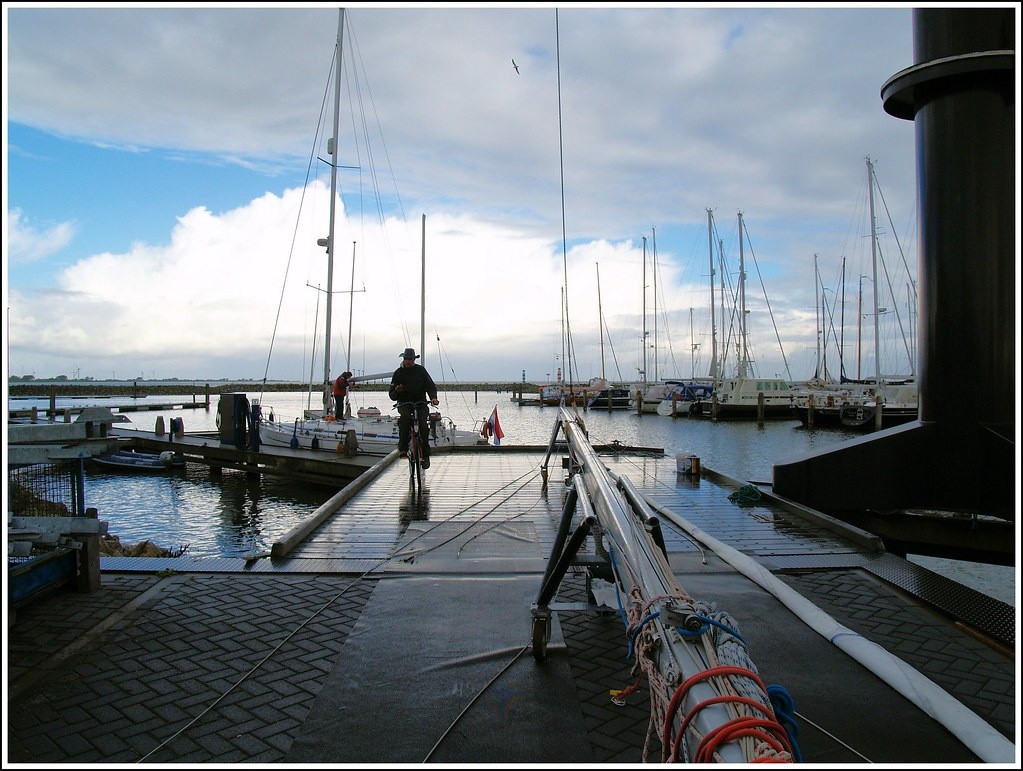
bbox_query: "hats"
[399,348,420,359]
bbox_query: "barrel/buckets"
[676,454,691,472]
[685,456,700,473]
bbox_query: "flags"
[489,405,505,445]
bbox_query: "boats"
[92,448,186,470]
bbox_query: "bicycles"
[391,400,441,496]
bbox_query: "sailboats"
[247,9,493,460]
[540,154,920,432]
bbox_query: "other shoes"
[340,417,346,420]
[421,456,430,469]
[398,451,407,458]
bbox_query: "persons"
[389,348,439,469]
[334,371,353,420]
